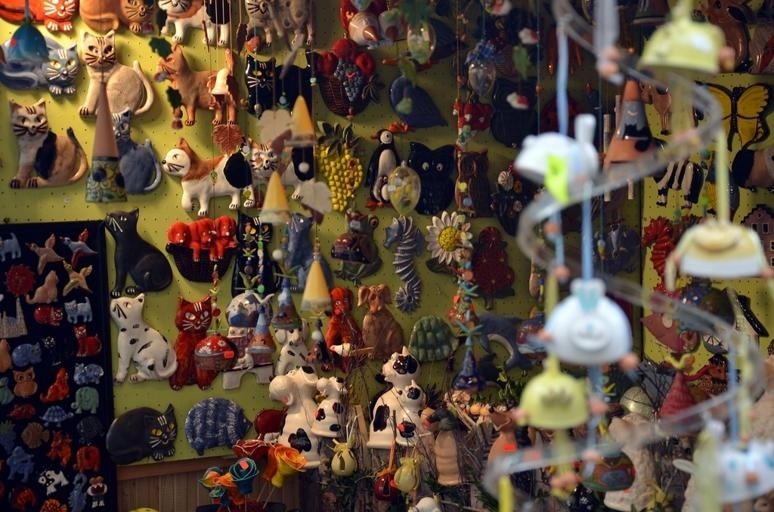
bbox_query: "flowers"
[194,437,308,512]
